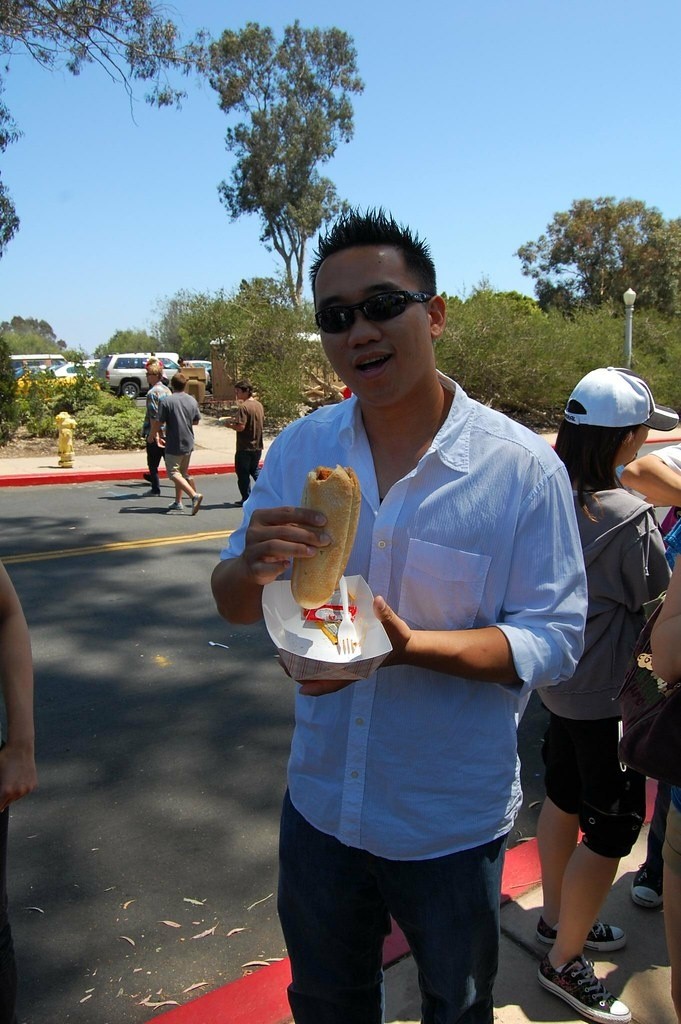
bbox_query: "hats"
[563,367,680,431]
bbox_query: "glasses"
[313,290,431,334]
[145,373,156,376]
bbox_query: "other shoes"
[235,500,243,504]
[144,474,152,482]
[143,489,159,497]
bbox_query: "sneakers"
[537,951,632,1024]
[631,859,663,906]
[168,499,183,510]
[192,493,203,516]
[535,914,626,952]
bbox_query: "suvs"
[98,354,210,401]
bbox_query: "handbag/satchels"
[613,591,681,788]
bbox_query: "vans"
[8,354,68,371]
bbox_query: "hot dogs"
[290,465,360,610]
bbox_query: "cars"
[184,360,213,394]
[14,359,101,380]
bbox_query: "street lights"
[623,288,636,369]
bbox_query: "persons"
[142,351,204,516]
[0,559,38,1024]
[211,214,589,1024]
[536,366,681,1024]
[224,380,264,507]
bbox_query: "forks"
[338,575,359,656]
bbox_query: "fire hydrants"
[53,412,77,468]
